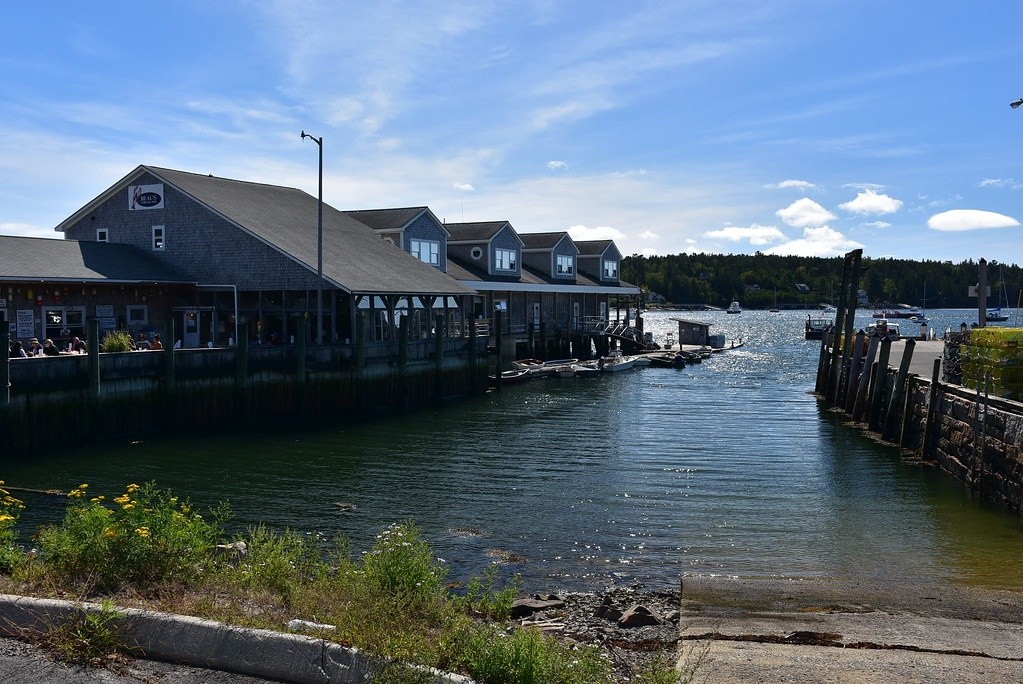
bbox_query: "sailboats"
[909,279,932,323]
[986,265,1013,322]
[770,286,780,312]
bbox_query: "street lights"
[301,130,323,347]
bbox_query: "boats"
[910,307,919,311]
[489,369,533,386]
[805,314,833,341]
[872,309,921,318]
[511,358,545,371]
[824,307,837,313]
[543,359,578,367]
[697,343,713,359]
[550,363,601,378]
[869,314,903,337]
[727,297,741,314]
[647,355,686,366]
[600,340,642,372]
[675,350,702,364]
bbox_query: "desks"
[59,351,79,355]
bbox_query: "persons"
[73,337,86,352]
[271,333,280,346]
[9,341,27,357]
[29,338,43,355]
[128,334,150,350]
[43,339,59,356]
[150,335,162,349]
